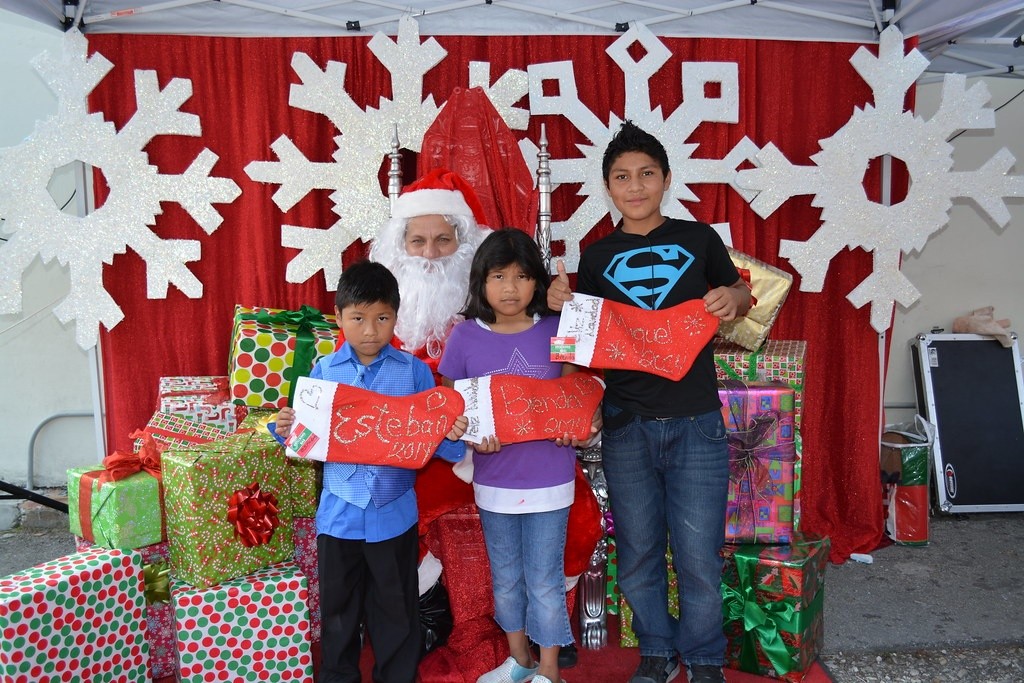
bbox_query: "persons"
[437,228,605,683]
[366,88,605,683]
[267,261,468,683]
[547,124,751,683]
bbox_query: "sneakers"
[686,665,725,683]
[630,656,681,683]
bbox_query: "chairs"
[387,122,610,649]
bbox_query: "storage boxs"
[601,242,831,683]
[906,325,1024,518]
[0,304,340,683]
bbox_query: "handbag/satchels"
[881,414,933,547]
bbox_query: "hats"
[392,174,490,229]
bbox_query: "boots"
[418,576,454,660]
[527,636,577,667]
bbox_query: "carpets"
[533,614,839,683]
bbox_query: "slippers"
[476,656,540,683]
[531,675,567,683]
